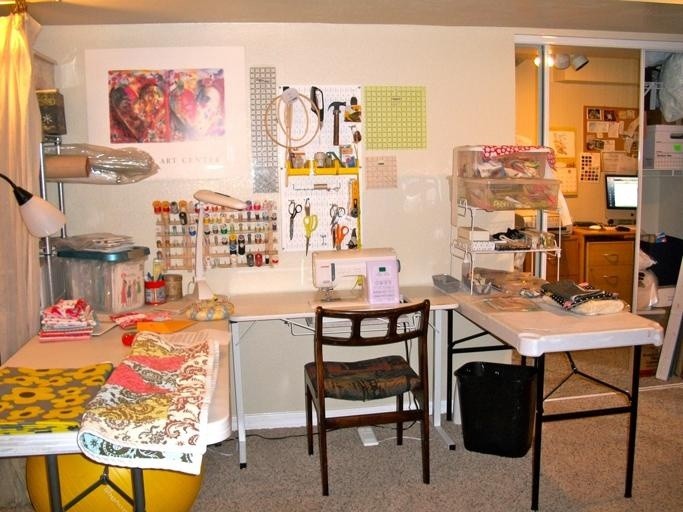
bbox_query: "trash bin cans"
[454,362,537,458]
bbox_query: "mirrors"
[514,45,641,407]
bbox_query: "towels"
[78,332,220,477]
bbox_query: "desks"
[433,278,664,507]
[1,293,230,512]
[225,284,461,470]
[569,222,635,306]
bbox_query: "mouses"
[616,226,631,231]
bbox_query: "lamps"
[0,170,66,241]
[536,47,591,73]
[191,184,248,303]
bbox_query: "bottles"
[314,153,333,170]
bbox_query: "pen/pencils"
[148,272,163,282]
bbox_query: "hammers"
[328,102,346,145]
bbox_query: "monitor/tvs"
[601,171,639,220]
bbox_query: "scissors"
[330,204,349,250]
[289,201,302,240]
[304,215,318,256]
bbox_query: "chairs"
[302,299,434,493]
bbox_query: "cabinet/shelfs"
[447,200,561,295]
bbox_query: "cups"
[162,274,182,303]
[145,276,167,306]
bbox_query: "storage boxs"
[36,228,149,311]
[644,125,682,171]
[446,146,560,212]
